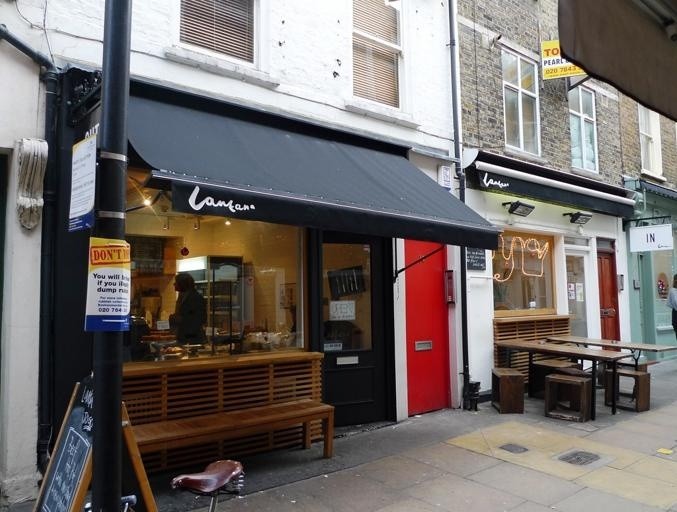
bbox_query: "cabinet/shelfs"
[175,251,246,341]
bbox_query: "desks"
[491,336,675,421]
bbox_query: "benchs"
[130,398,337,482]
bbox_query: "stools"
[487,358,662,422]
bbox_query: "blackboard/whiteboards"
[122,403,157,511]
[32,381,93,512]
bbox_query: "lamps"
[500,199,536,218]
[562,209,593,228]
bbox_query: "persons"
[169,273,208,345]
[667,274,677,342]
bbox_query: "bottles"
[146,310,152,328]
